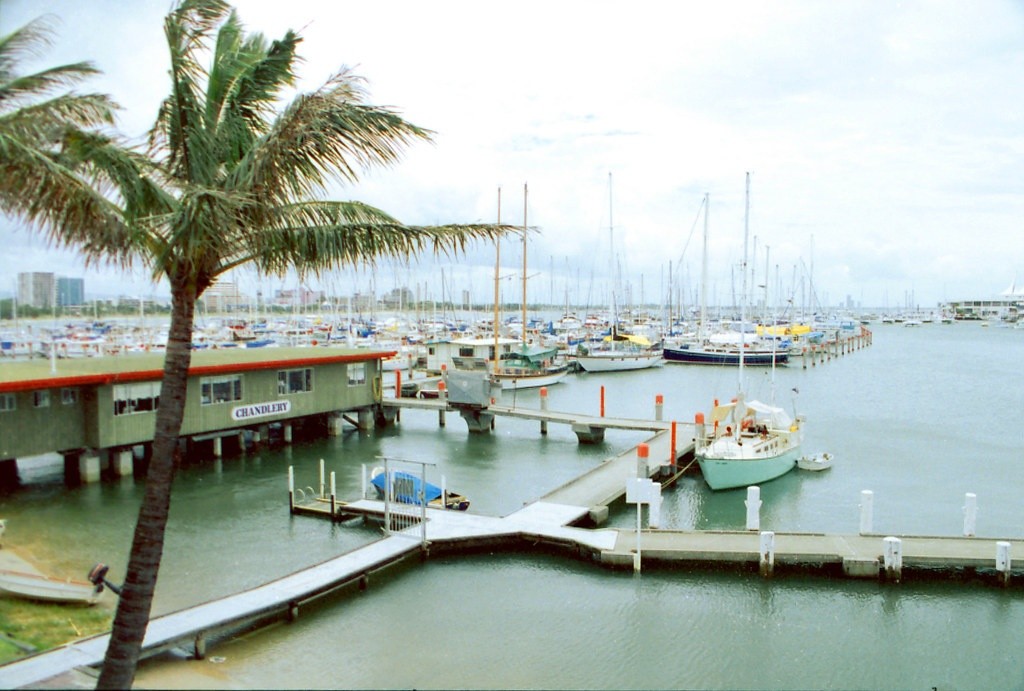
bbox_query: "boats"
[1,534,108,604]
[796,451,835,472]
[371,465,471,513]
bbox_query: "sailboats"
[692,169,807,492]
[0,171,1024,396]
